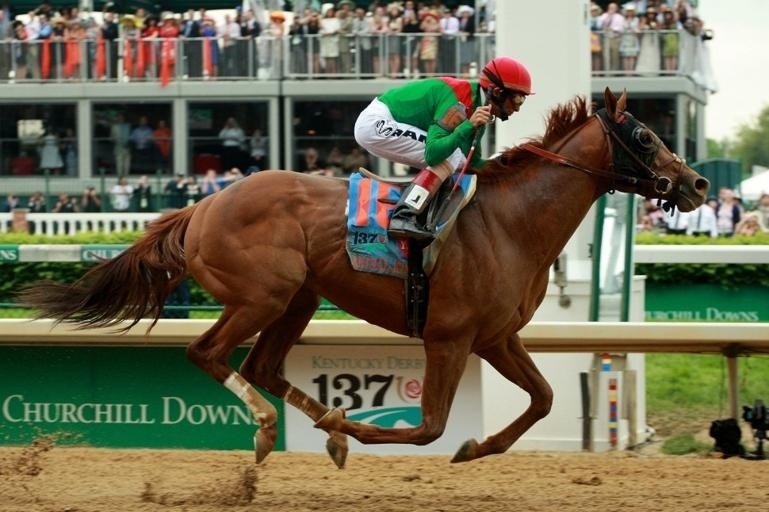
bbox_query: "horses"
[14,83,712,472]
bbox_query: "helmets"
[478,55,535,95]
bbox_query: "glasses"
[499,92,526,105]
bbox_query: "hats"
[591,1,689,14]
[49,0,480,28]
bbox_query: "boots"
[387,168,443,240]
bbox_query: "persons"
[354,57,532,242]
[589,1,692,76]
[303,145,369,176]
[5,168,246,214]
[651,189,742,238]
[41,116,171,175]
[219,116,268,172]
